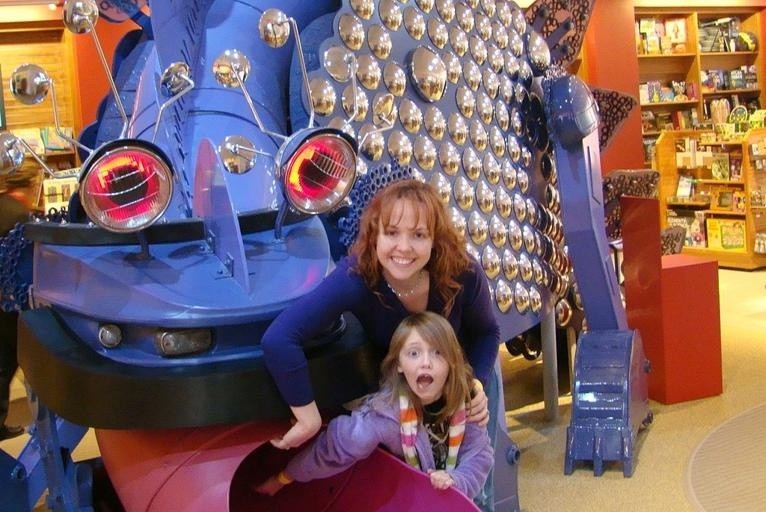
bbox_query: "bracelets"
[277,471,289,487]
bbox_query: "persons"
[250,310,496,505]
[1,160,45,442]
[259,180,502,511]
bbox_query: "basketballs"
[736,33,756,51]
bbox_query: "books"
[675,137,744,182]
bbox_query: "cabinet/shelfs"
[654,126,765,271]
[634,6,766,165]
